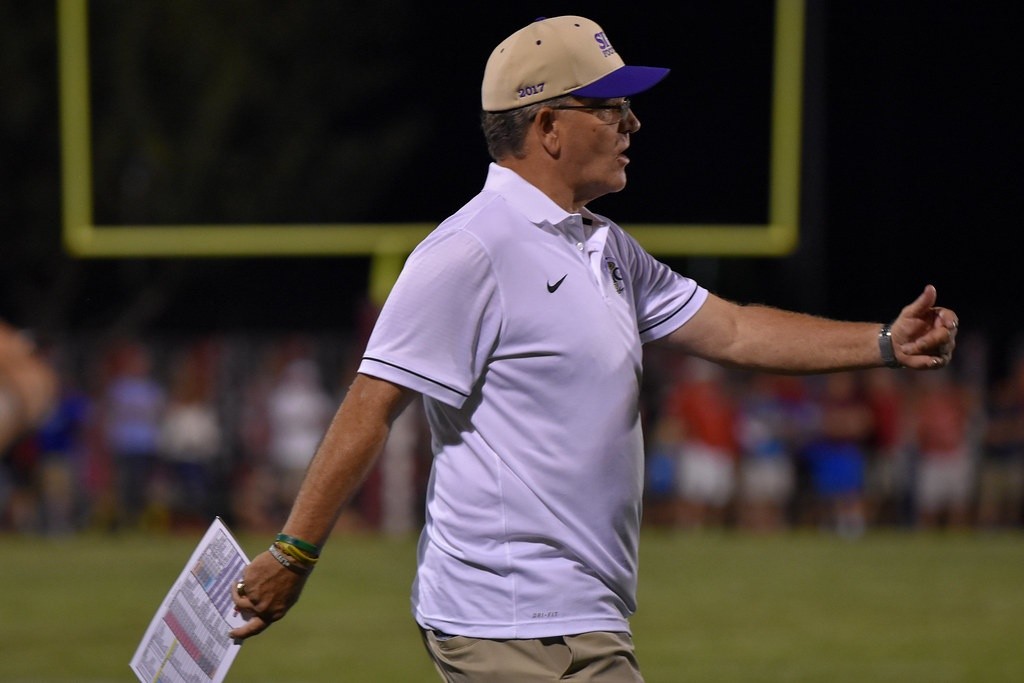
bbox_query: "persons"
[1,329,981,533]
[228,14,960,683]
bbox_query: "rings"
[236,577,246,594]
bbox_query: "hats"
[482,15,669,111]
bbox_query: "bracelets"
[878,324,896,363]
[269,535,318,575]
